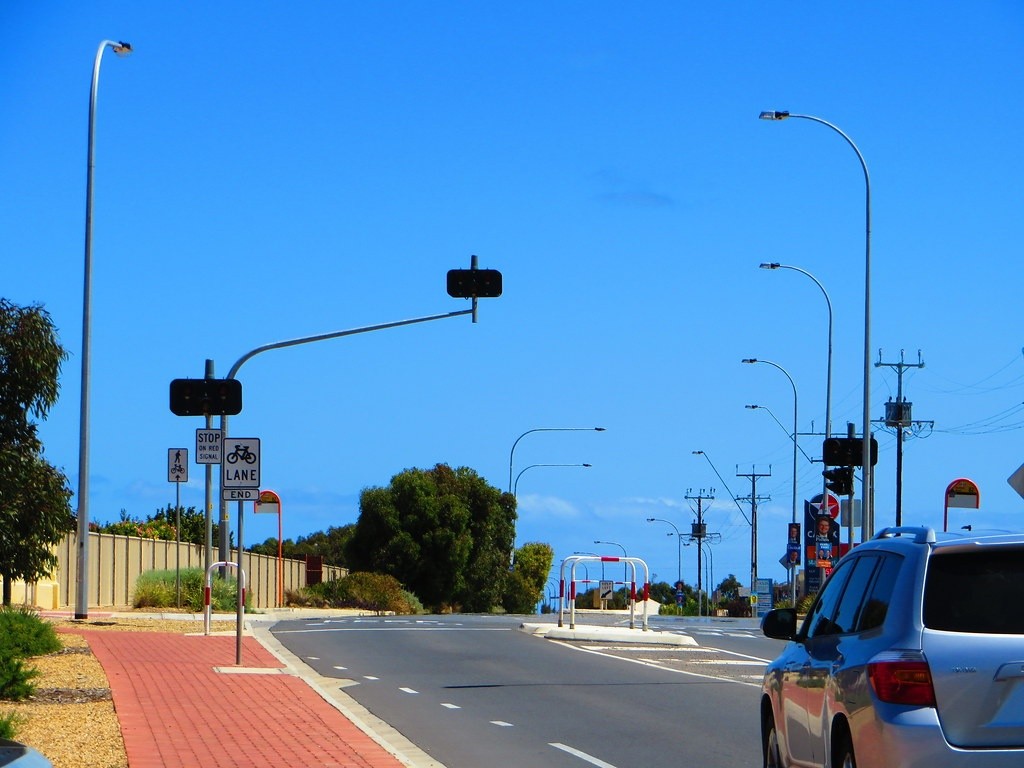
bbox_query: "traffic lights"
[822,438,878,466]
[821,468,856,496]
[759,110,871,545]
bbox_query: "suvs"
[758,522,1024,768]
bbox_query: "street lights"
[690,448,758,619]
[593,540,627,610]
[540,559,588,615]
[71,37,135,623]
[745,403,828,587]
[646,518,683,616]
[573,551,605,610]
[758,262,833,514]
[741,356,799,638]
[508,463,593,572]
[508,426,608,573]
